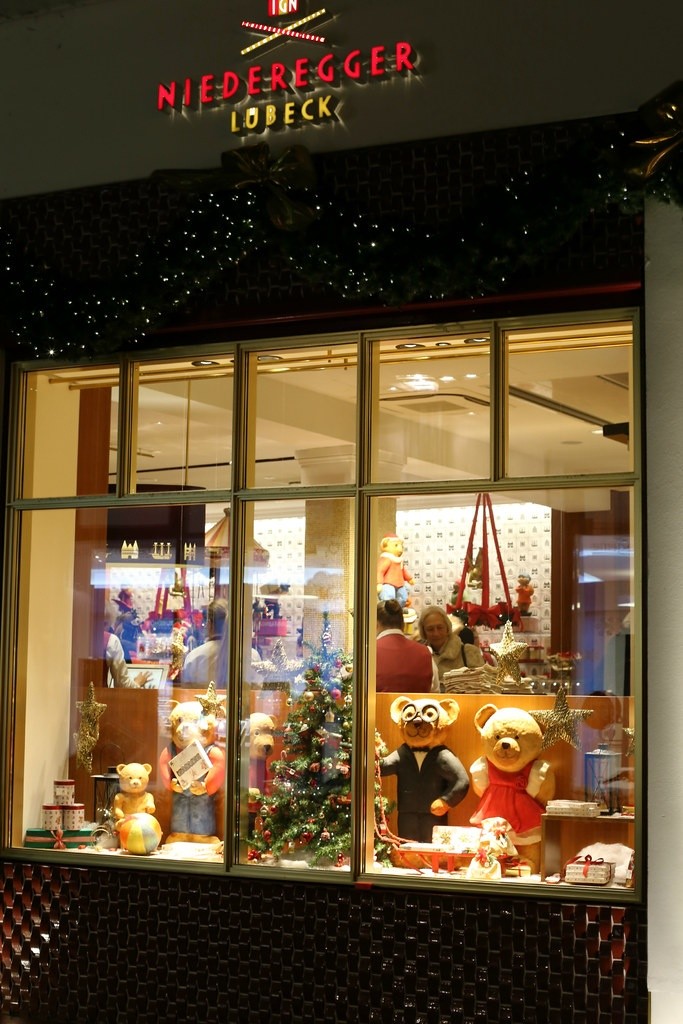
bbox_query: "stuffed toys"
[159,699,226,844]
[376,532,414,607]
[469,704,555,874]
[515,572,534,617]
[114,763,155,832]
[246,712,277,835]
[375,696,470,843]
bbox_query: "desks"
[539,813,635,881]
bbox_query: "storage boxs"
[168,741,214,792]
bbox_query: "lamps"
[205,508,270,566]
[583,744,622,817]
[90,742,125,825]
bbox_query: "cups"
[63,803,85,830]
[53,779,75,806]
[41,803,63,830]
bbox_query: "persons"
[103,631,153,688]
[458,628,498,667]
[417,606,485,681]
[182,600,263,683]
[375,599,441,693]
